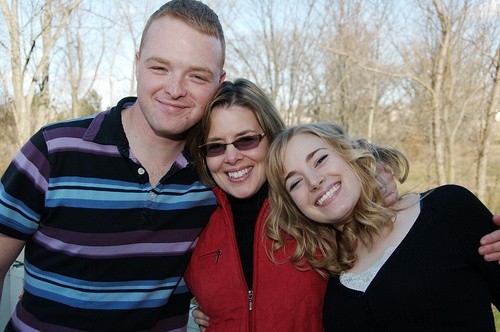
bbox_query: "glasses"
[196,129,267,158]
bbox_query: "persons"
[178,73,500,331]
[0,0,233,332]
[191,120,500,331]
